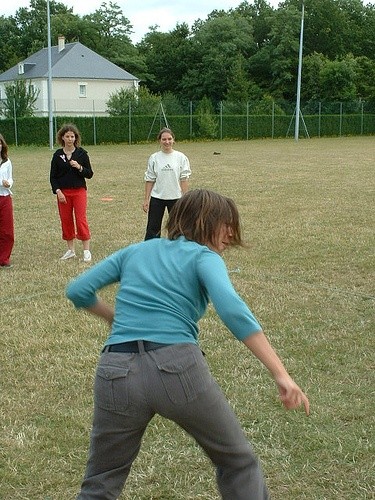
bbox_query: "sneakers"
[60,249,76,260]
[84,250,92,262]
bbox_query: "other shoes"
[1,263,14,268]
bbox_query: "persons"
[143,128,192,242]
[65,189,311,500]
[0,134,14,269]
[50,123,94,261]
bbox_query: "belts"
[102,340,169,353]
